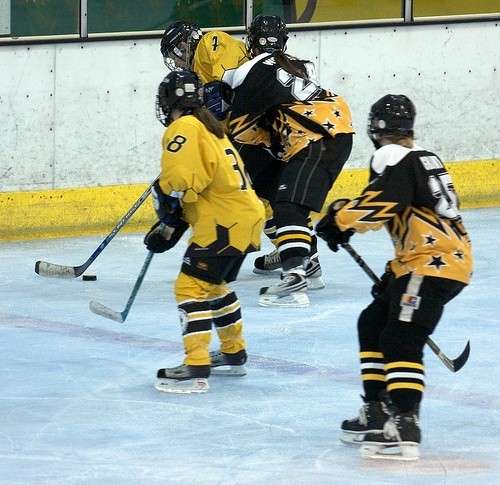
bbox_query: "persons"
[316,95,474,461]
[227,14,354,306]
[145,70,266,392]
[160,21,285,274]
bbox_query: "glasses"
[159,20,203,65]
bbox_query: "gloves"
[314,198,355,253]
[143,216,189,254]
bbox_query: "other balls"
[83,275,96,281]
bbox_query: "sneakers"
[338,398,393,446]
[257,272,310,309]
[205,349,248,376]
[356,410,422,463]
[153,363,212,394]
[251,246,284,275]
[279,255,325,291]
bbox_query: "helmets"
[245,13,289,52]
[152,69,203,126]
[367,92,416,137]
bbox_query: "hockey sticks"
[34,171,163,277]
[343,240,470,372]
[89,222,167,323]
